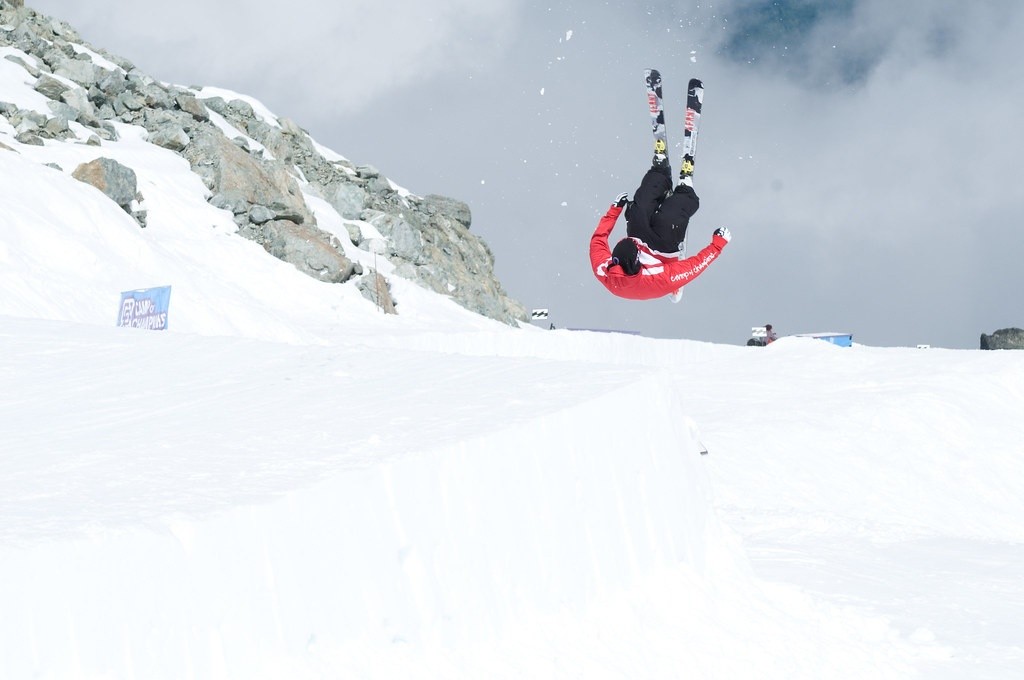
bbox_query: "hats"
[612,238,641,275]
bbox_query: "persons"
[590,142,732,300]
[761,324,775,345]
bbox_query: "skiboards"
[643,66,704,305]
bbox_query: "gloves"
[714,227,732,242]
[612,192,629,207]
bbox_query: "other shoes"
[652,139,669,167]
[679,154,694,180]
